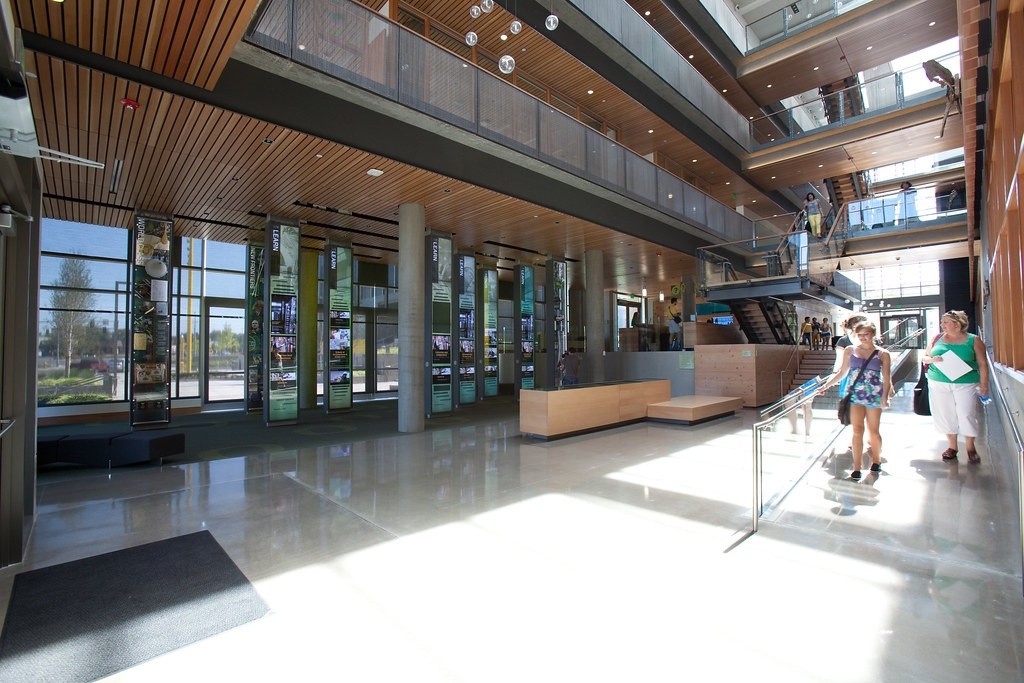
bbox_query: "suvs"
[82,357,109,370]
[39,340,55,357]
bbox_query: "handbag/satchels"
[923,334,942,372]
[674,316,682,323]
[838,396,851,425]
[914,363,931,416]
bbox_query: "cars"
[107,358,126,372]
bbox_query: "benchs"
[37,431,187,473]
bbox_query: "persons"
[801,317,812,350]
[896,181,921,225]
[803,193,824,238]
[274,349,286,387]
[832,313,893,480]
[631,312,640,328]
[784,396,814,445]
[667,297,681,349]
[820,318,832,350]
[811,317,820,350]
[922,310,989,463]
[557,347,579,386]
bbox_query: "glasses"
[947,311,960,320]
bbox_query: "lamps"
[641,277,649,299]
[659,288,664,303]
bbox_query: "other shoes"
[942,447,958,459]
[967,449,980,462]
[851,471,862,480]
[871,463,882,472]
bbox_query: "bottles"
[975,386,992,405]
